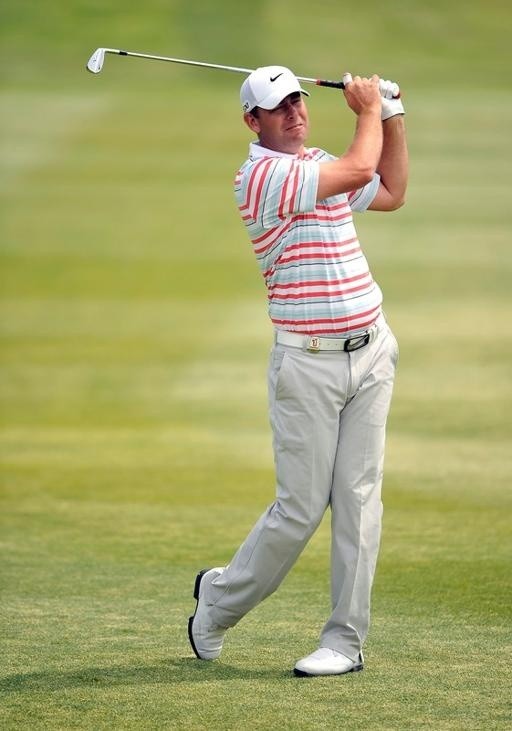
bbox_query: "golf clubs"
[86,47,402,100]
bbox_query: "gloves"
[369,78,406,122]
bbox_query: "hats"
[240,66,310,113]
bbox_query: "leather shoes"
[294,648,364,677]
[189,568,228,659]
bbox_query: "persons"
[187,64,410,677]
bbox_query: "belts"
[276,312,385,352]
